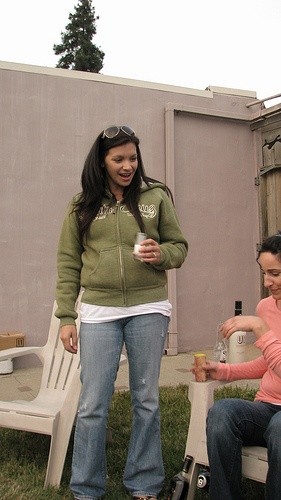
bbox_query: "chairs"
[165,330,269,500]
[0,291,127,488]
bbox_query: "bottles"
[214,319,227,363]
[164,455,193,500]
[229,300,246,365]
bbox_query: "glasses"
[102,125,136,139]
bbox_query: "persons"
[191,231,281,500]
[54,126,189,500]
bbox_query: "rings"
[153,252,156,257]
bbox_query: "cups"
[133,233,157,259]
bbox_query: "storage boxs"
[0,333,26,351]
